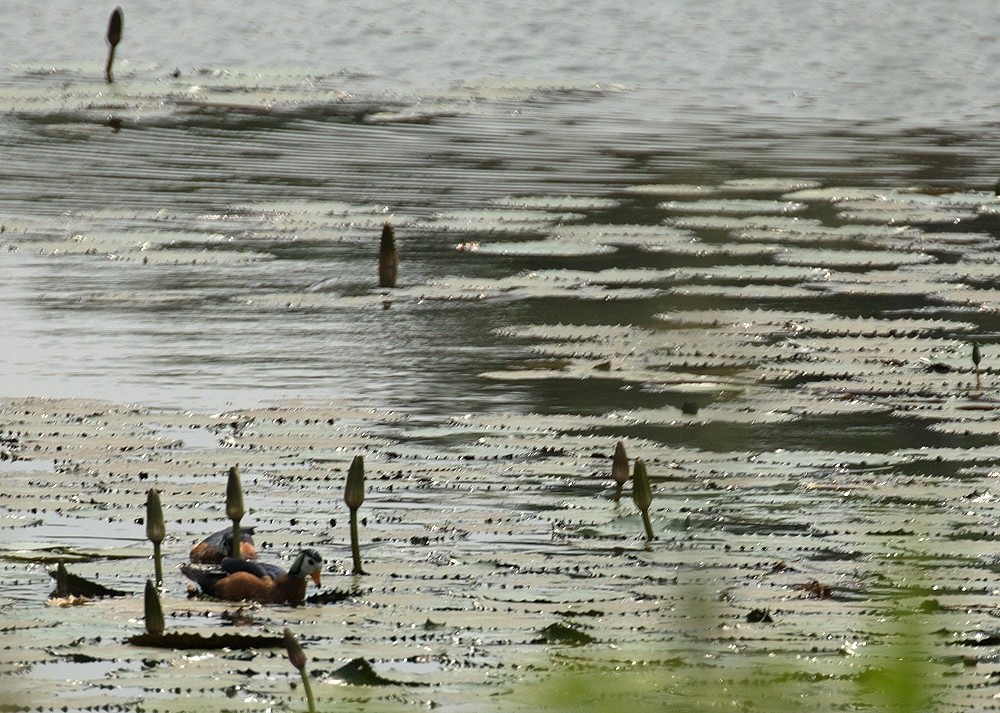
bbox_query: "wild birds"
[179,549,323,606]
[189,526,259,564]
